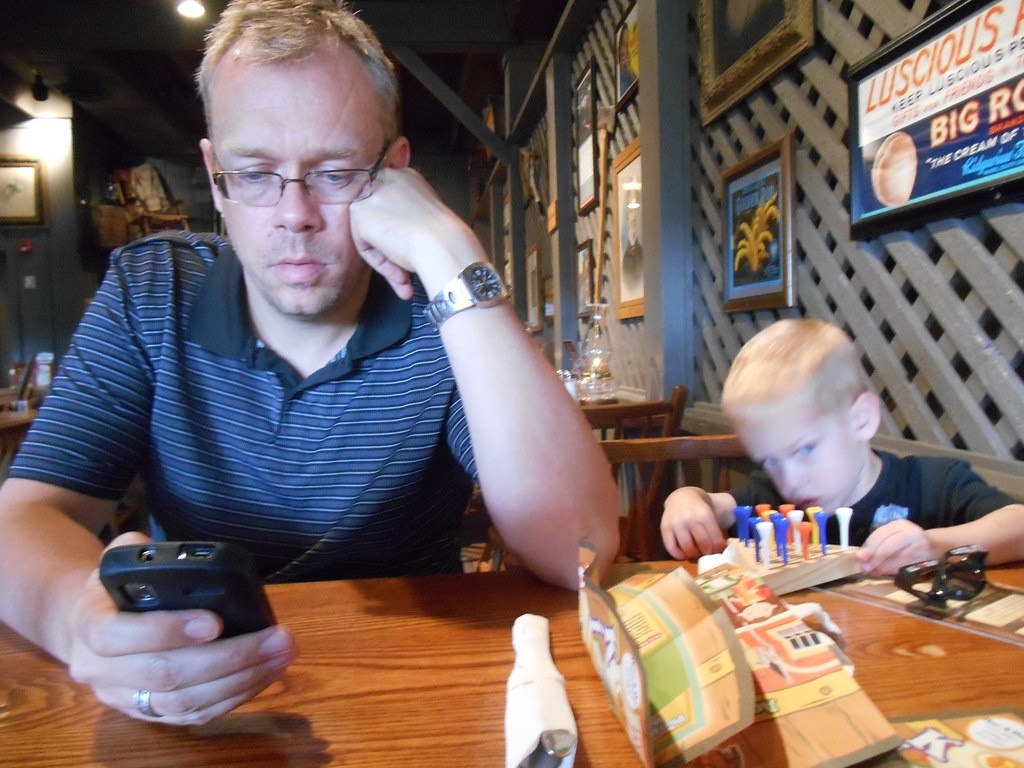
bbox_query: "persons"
[660,319,1024,576]
[0,0,621,727]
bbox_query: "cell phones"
[99,542,278,643]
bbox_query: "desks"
[0,554,1024,768]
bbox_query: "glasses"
[212,153,386,207]
[892,545,990,607]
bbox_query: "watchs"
[424,260,514,331]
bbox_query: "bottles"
[576,303,619,400]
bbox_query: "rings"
[133,691,163,720]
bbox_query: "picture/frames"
[843,0,1024,242]
[573,55,599,217]
[525,243,544,333]
[611,136,644,320]
[613,0,639,115]
[697,0,812,129]
[717,133,796,314]
[0,153,52,236]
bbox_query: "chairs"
[577,384,755,564]
